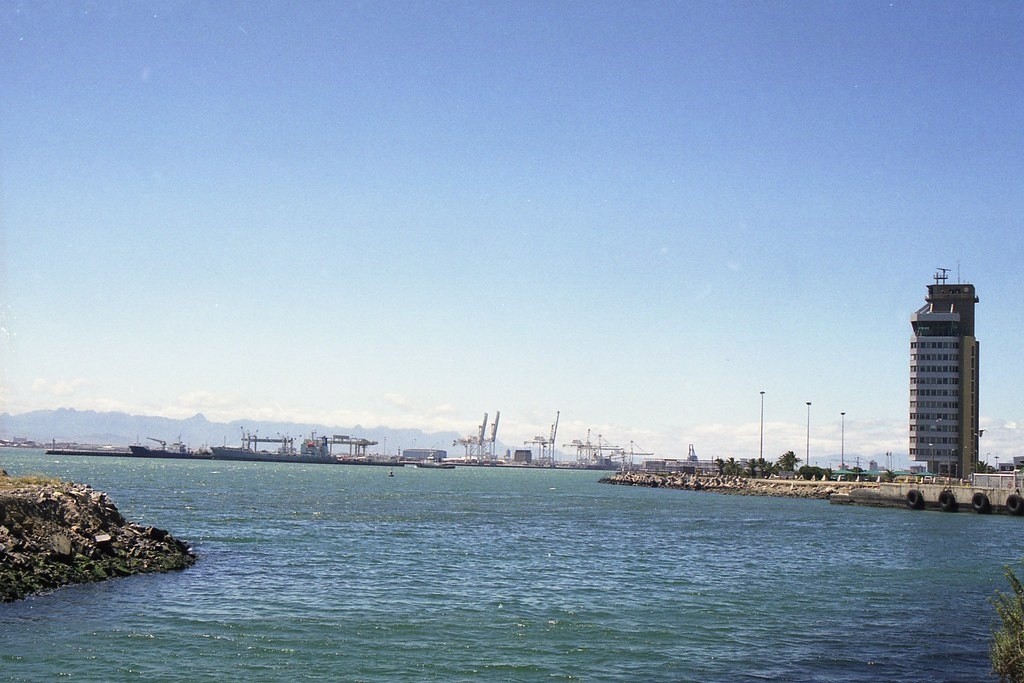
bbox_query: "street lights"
[759,390,766,459]
[929,444,935,479]
[947,448,955,483]
[840,412,846,469]
[805,401,812,465]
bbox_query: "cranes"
[451,411,504,466]
[564,428,655,472]
[525,410,562,468]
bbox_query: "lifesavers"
[1006,495,1023,513]
[938,492,954,510]
[972,493,989,512]
[1008,484,1012,488]
[907,491,923,508]
